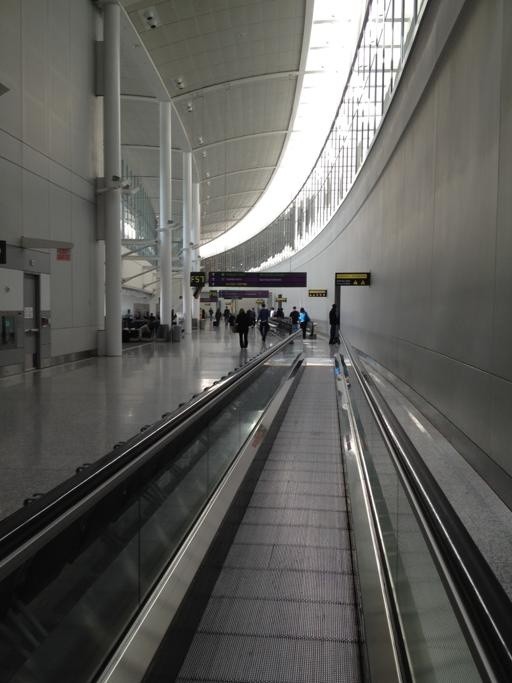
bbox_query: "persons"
[201,303,284,350]
[289,306,300,333]
[126,308,177,326]
[298,308,310,339]
[329,304,340,344]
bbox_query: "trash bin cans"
[201,318,210,329]
[172,325,182,342]
